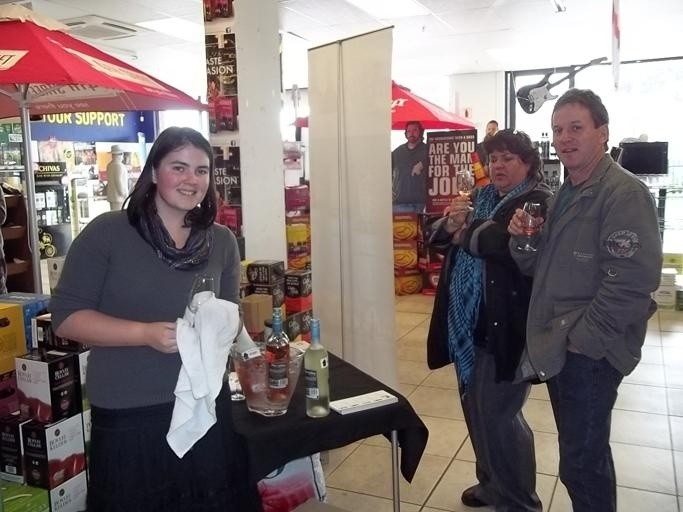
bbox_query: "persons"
[476,121,499,166]
[507,90,663,512]
[423,129,555,512]
[51,125,244,512]
[0,186,7,295]
[107,144,130,211]
[392,122,429,203]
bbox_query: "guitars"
[517,57,607,113]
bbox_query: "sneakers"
[461,478,498,508]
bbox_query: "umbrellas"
[0,0,211,295]
[288,79,475,129]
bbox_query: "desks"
[222,346,403,512]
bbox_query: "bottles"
[300,319,331,419]
[264,307,290,400]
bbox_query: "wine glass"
[188,272,213,316]
[515,200,541,254]
[455,170,478,212]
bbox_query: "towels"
[162,291,258,461]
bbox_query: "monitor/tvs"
[621,142,669,176]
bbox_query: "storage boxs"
[0,283,111,512]
[647,266,683,315]
[214,179,446,339]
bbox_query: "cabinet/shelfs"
[0,190,33,294]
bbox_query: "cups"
[232,342,305,418]
